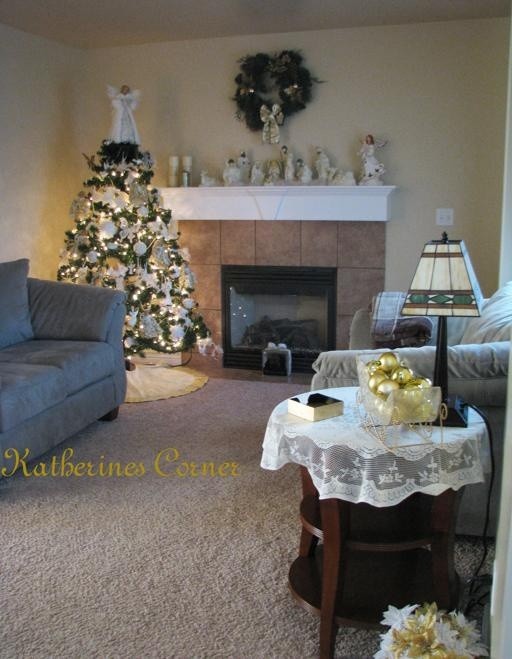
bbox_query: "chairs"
[304,275,511,545]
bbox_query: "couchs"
[1,254,130,480]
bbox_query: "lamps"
[401,231,487,427]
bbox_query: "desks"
[263,389,486,658]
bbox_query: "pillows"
[1,254,39,353]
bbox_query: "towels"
[367,290,433,347]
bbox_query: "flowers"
[373,604,489,658]
